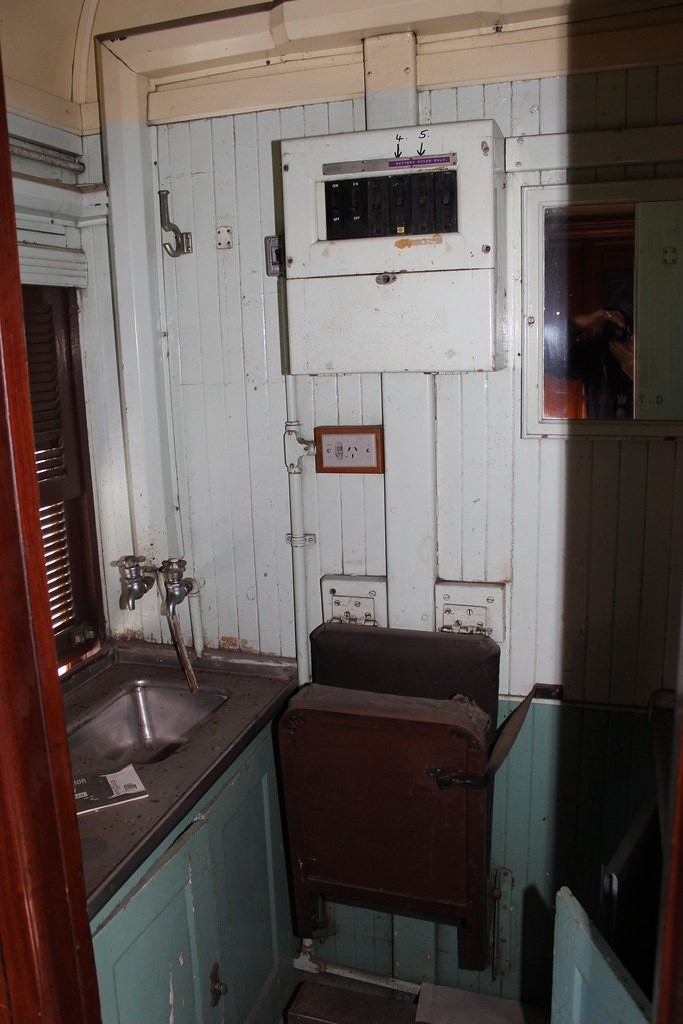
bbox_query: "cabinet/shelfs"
[93,722,300,1024]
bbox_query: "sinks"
[67,679,230,765]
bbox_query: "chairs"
[272,619,502,972]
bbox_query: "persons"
[566,304,634,420]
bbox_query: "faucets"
[116,554,150,611]
[160,558,192,617]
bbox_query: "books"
[71,764,149,814]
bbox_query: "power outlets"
[314,425,385,474]
